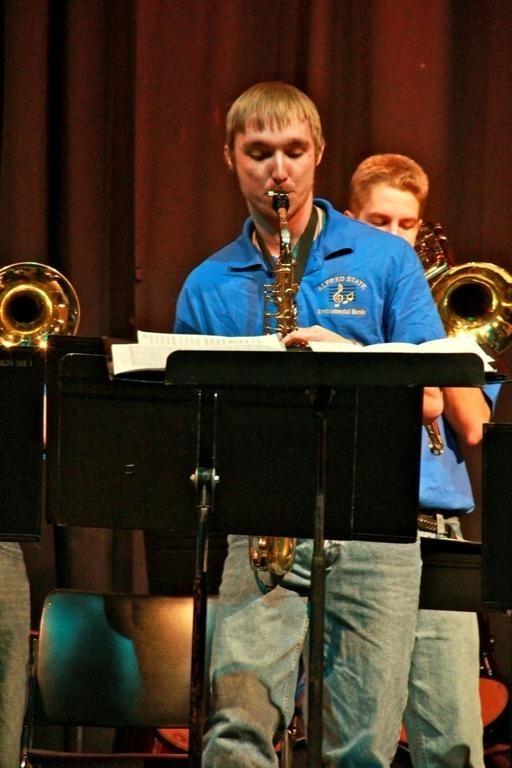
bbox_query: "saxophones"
[250,191,299,578]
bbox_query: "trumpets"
[0,262,80,354]
[414,221,512,454]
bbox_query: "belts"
[417,512,458,540]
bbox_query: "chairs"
[400,607,510,768]
[155,730,191,759]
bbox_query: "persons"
[170,81,445,768]
[337,148,504,768]
[0,542,30,765]
